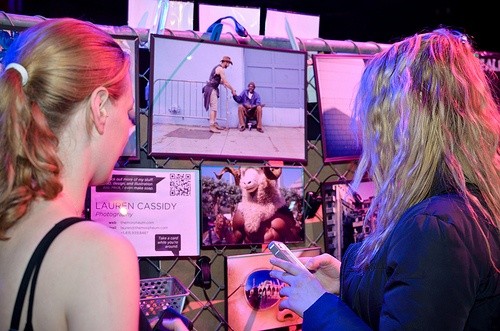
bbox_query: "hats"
[221,56,233,65]
[248,82,255,88]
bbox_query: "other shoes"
[216,126,224,130]
[240,126,245,131]
[210,130,220,133]
[257,127,264,133]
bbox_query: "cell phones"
[268,241,308,271]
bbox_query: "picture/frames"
[146,34,309,166]
[87,168,206,259]
[323,178,384,257]
[0,25,141,162]
[197,164,307,251]
[223,247,321,331]
[316,51,385,165]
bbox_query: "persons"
[0,18,189,331]
[270,29,500,331]
[202,56,236,133]
[232,82,264,133]
